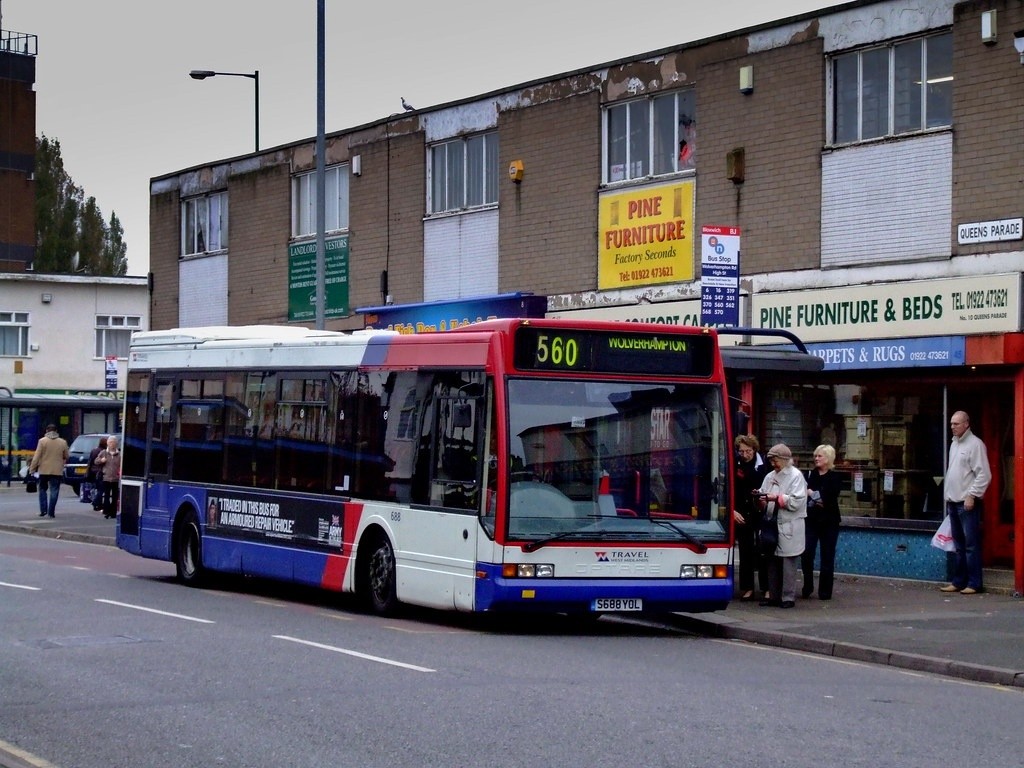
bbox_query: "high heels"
[759,596,772,605]
[741,590,755,601]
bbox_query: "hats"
[766,444,792,459]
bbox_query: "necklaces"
[802,445,843,599]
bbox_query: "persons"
[86,436,121,518]
[752,444,807,608]
[473,430,534,493]
[722,435,770,601]
[29,424,69,518]
[5,426,18,476]
[941,412,993,594]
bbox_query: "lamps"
[981,9,997,45]
[739,65,754,94]
[352,151,361,176]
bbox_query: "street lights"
[190,66,261,153]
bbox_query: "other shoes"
[39,511,47,516]
[50,515,55,518]
[960,587,983,593]
[818,593,831,600]
[803,588,813,599]
[782,601,796,608]
[940,584,960,591]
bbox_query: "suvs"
[62,433,110,497]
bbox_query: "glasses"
[735,449,754,454]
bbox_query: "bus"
[114,316,755,627]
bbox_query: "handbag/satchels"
[80,482,96,503]
[757,505,778,554]
[96,470,103,481]
[930,514,957,551]
[26,474,38,492]
